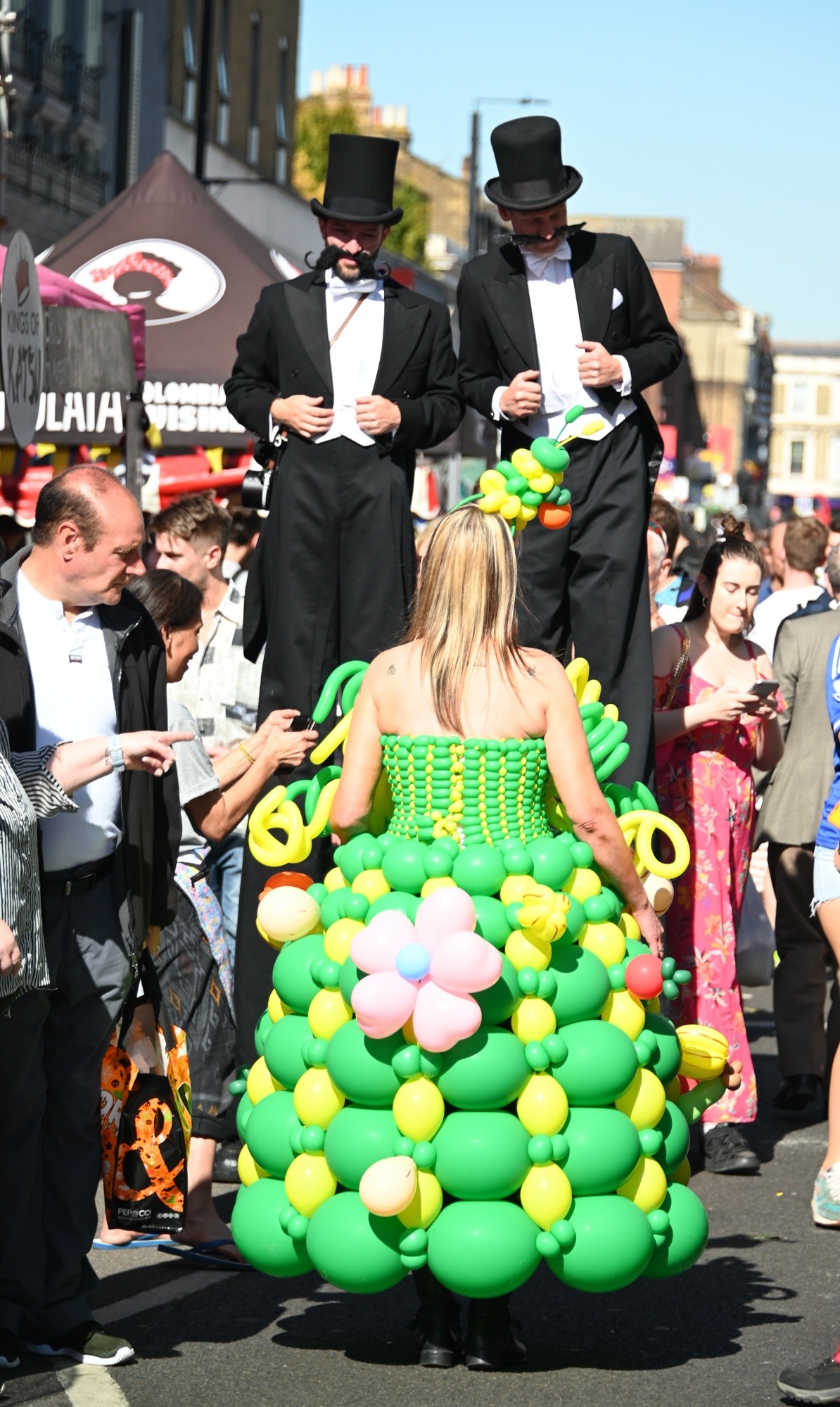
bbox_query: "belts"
[38,861,112,899]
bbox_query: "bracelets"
[107,734,125,772]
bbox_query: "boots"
[411,1288,462,1366]
[464,1292,528,1369]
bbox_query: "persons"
[327,507,665,1368]
[650,511,840,1404]
[0,132,466,1367]
[454,115,684,788]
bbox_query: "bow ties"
[328,276,377,297]
[524,239,572,277]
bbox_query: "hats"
[485,116,582,211]
[311,134,403,228]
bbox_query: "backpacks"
[773,593,834,659]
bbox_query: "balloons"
[446,405,605,531]
[230,660,744,1299]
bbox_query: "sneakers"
[0,1329,21,1369]
[777,1357,840,1404]
[26,1321,135,1366]
[687,1125,760,1173]
[811,1161,840,1227]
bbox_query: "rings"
[658,949,664,952]
[662,942,664,946]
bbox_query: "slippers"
[158,1240,251,1271]
[92,1232,184,1250]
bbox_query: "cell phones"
[741,680,779,711]
[286,715,315,732]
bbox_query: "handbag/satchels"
[737,873,778,987]
[102,949,191,1233]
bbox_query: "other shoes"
[772,1074,818,1110]
[211,1138,244,1183]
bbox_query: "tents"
[34,151,498,511]
[0,242,147,564]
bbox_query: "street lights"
[455,96,560,460]
